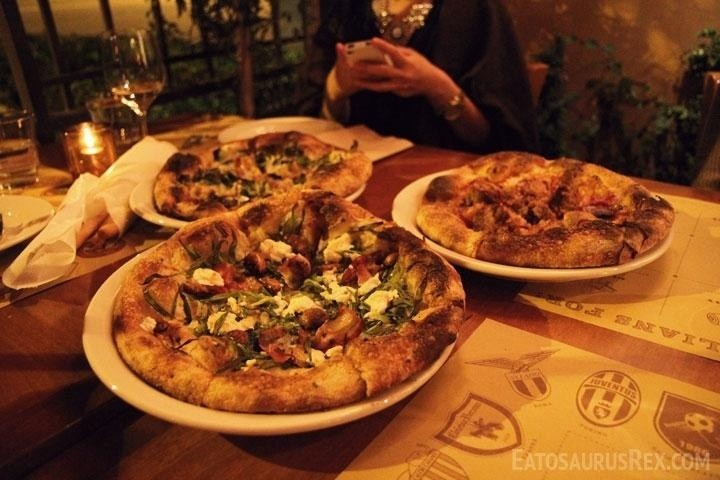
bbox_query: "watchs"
[436,86,465,124]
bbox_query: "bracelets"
[324,65,349,103]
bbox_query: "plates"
[132,165,368,231]
[390,165,674,283]
[84,216,469,435]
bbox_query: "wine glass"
[98,26,166,139]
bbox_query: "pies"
[417,151,674,269]
[113,188,467,413]
[153,130,373,223]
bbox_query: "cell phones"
[344,39,394,67]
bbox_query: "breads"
[76,205,119,254]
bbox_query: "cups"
[1,110,38,185]
[65,120,113,178]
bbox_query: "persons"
[300,0,538,157]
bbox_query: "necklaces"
[371,0,434,45]
